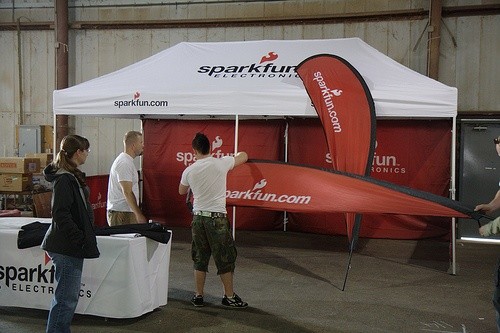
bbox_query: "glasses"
[80,149,91,153]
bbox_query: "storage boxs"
[0,152,53,192]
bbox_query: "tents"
[53,38,457,274]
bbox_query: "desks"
[0,217,172,319]
[0,189,51,211]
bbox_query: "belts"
[194,210,226,219]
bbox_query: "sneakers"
[222,294,249,308]
[191,296,206,308]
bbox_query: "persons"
[474,137,500,311]
[106,129,146,226]
[38,133,100,332]
[178,132,248,308]
